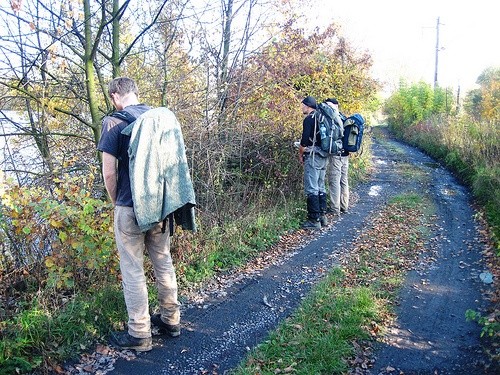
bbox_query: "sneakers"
[109,331,153,351]
[151,313,180,337]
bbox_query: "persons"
[298,96,328,229]
[324,99,349,217]
[97,78,180,353]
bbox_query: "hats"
[326,98,338,104]
[302,96,316,109]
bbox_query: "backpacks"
[309,103,344,155]
[338,112,364,151]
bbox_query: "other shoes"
[325,208,340,216]
[339,207,348,213]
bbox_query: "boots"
[318,192,328,226]
[302,195,321,229]
